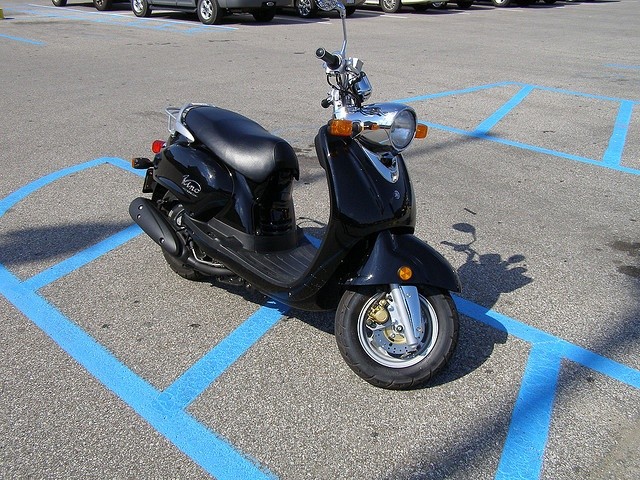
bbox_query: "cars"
[365,0,449,14]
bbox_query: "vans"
[281,0,366,18]
[132,0,293,27]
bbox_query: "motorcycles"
[128,0,462,389]
